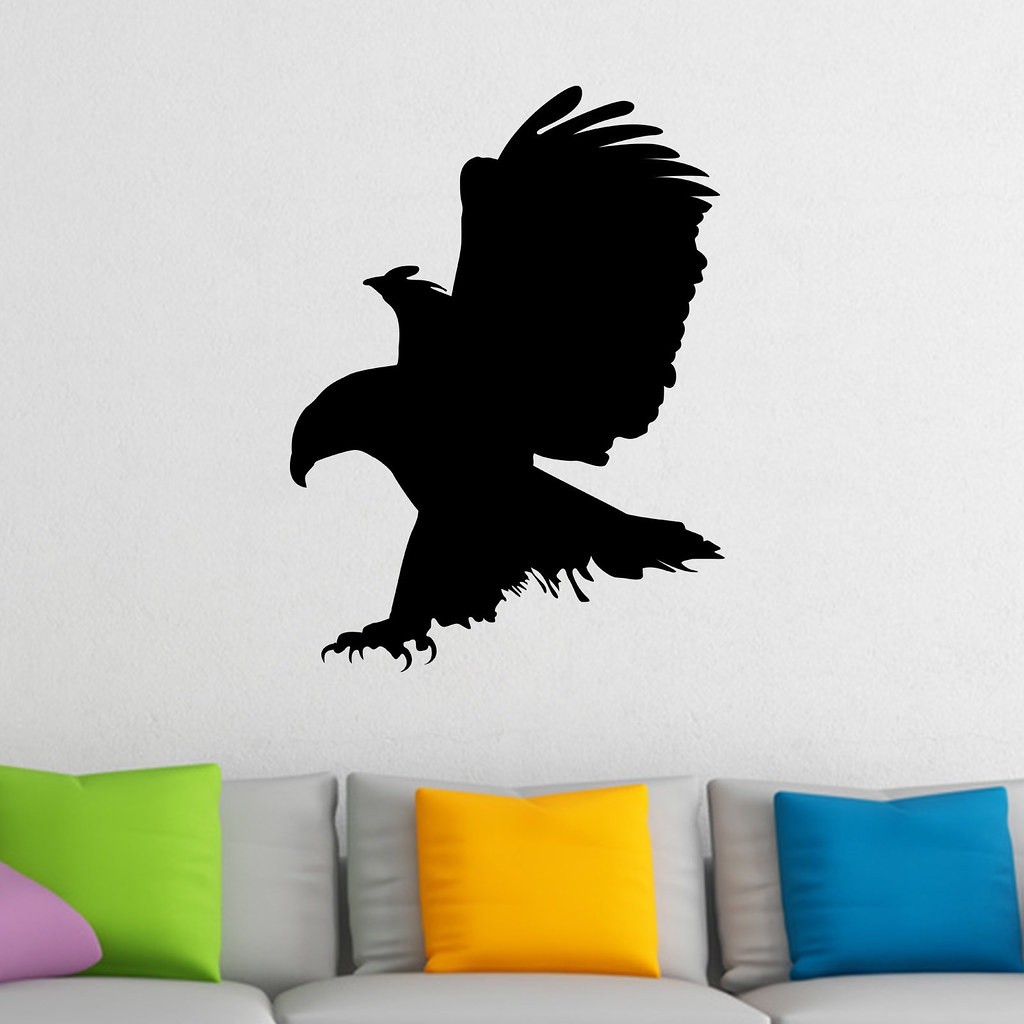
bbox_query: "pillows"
[0,762,223,984]
[0,861,103,983]
[414,783,660,979]
[774,786,1024,981]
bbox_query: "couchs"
[0,761,1024,1024]
